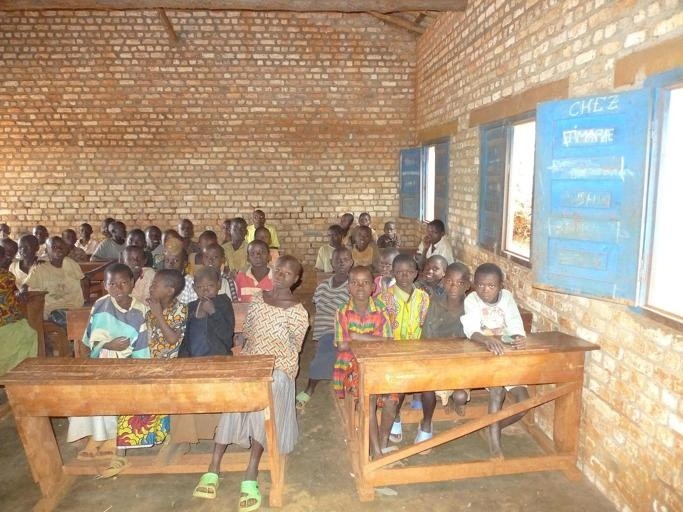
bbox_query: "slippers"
[414,421,433,455]
[238,480,261,512]
[296,391,311,409]
[77,438,131,479]
[193,472,221,499]
[389,422,403,443]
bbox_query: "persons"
[2,208,533,512]
